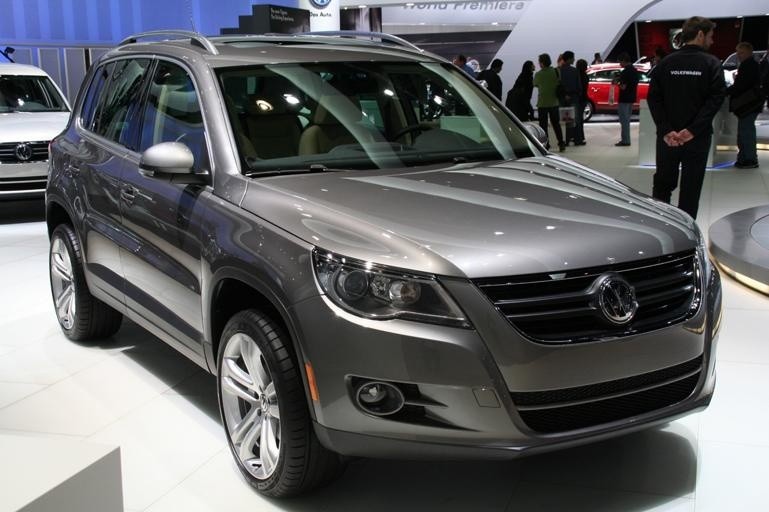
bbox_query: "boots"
[44,31,724,500]
[0,61,72,203]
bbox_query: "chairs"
[545,142,551,150]
[575,141,586,146]
[615,140,632,147]
[734,161,760,169]
[566,140,570,146]
[558,144,566,152]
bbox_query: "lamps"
[297,94,375,156]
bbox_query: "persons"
[647,17,726,221]
[533,54,566,154]
[649,44,667,65]
[557,55,565,67]
[554,51,586,146]
[612,53,639,146]
[505,60,536,122]
[592,53,603,65]
[725,42,766,169]
[572,60,590,141]
[475,58,503,101]
[452,54,476,81]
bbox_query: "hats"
[721,51,767,88]
[582,55,653,124]
